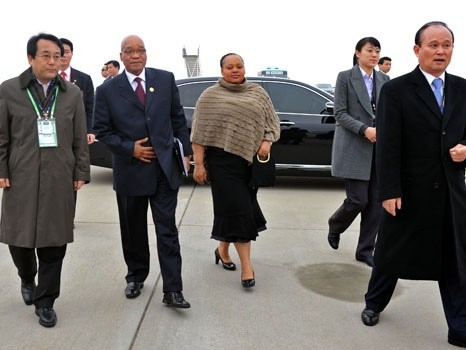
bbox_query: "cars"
[316,84,336,95]
[89,76,341,178]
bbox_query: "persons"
[189,53,281,288]
[327,37,389,266]
[106,60,120,78]
[0,33,90,327]
[362,22,466,346]
[101,63,111,82]
[93,34,194,306]
[378,57,392,74]
[58,37,95,145]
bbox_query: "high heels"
[241,271,256,288]
[215,247,236,271]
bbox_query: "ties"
[134,78,145,107]
[61,72,66,79]
[432,79,444,114]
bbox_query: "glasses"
[123,49,147,55]
[102,68,108,72]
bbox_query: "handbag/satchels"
[249,151,277,187]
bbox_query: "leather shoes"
[21,279,36,305]
[362,256,375,267]
[162,292,191,308]
[362,307,379,326]
[328,220,340,249]
[35,306,57,328]
[125,282,144,299]
